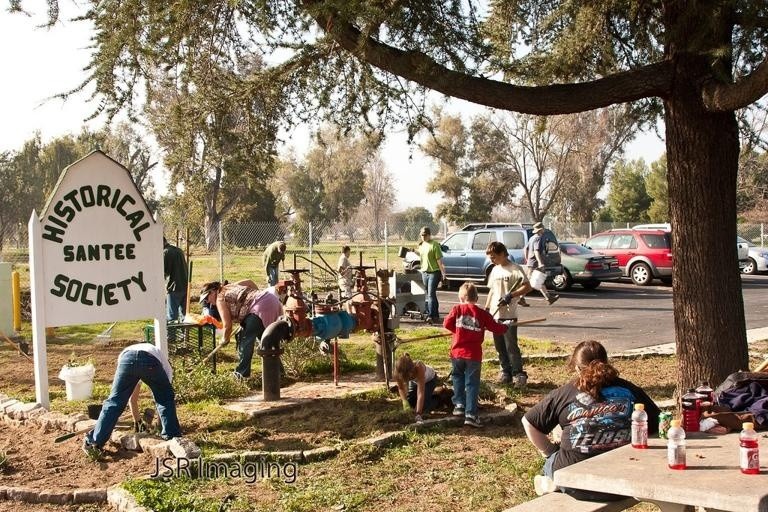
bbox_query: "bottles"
[202,306,210,322]
[178,306,184,323]
[665,417,691,471]
[737,420,760,475]
[680,381,715,432]
[631,403,648,449]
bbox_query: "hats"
[197,286,218,304]
[531,222,546,234]
[420,225,431,236]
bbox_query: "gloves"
[400,399,413,413]
[133,419,146,433]
[498,294,513,306]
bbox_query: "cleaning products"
[682,380,714,430]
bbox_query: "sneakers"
[517,298,530,307]
[495,369,530,389]
[547,294,560,305]
[452,404,486,428]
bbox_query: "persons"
[521,339,662,503]
[84,341,183,449]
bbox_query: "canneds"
[658,412,672,440]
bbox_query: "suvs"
[400,222,562,294]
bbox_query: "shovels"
[175,261,194,356]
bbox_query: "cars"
[577,223,767,287]
[546,239,622,292]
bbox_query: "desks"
[145,320,216,373]
[554,429,768,512]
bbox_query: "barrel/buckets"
[62,363,95,402]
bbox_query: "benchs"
[499,488,640,511]
